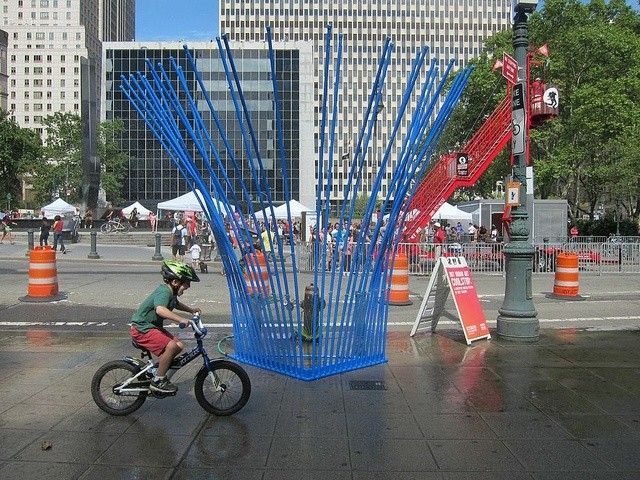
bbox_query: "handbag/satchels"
[0,221,7,232]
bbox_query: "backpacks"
[172,226,185,247]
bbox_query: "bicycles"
[101,217,131,234]
[91,311,250,416]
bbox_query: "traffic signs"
[502,51,518,84]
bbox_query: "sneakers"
[196,269,202,273]
[150,375,178,392]
[62,249,66,254]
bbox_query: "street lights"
[613,203,626,272]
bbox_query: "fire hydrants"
[290,284,326,342]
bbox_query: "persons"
[107,205,116,221]
[273,219,301,244]
[309,218,386,274]
[131,260,201,394]
[40,217,50,247]
[434,222,445,268]
[171,217,188,264]
[184,218,194,251]
[129,207,138,228]
[256,222,275,259]
[85,209,92,230]
[149,211,157,233]
[571,225,578,240]
[53,215,64,253]
[445,220,501,245]
[1,212,18,244]
[188,215,197,248]
[420,223,429,252]
[407,226,422,274]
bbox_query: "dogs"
[199,261,207,273]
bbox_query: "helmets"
[161,260,200,284]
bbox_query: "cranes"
[366,54,599,273]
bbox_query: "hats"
[434,223,441,228]
[186,218,192,222]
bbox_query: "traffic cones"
[18,245,69,303]
[377,251,414,306]
[545,251,592,301]
[243,249,269,298]
